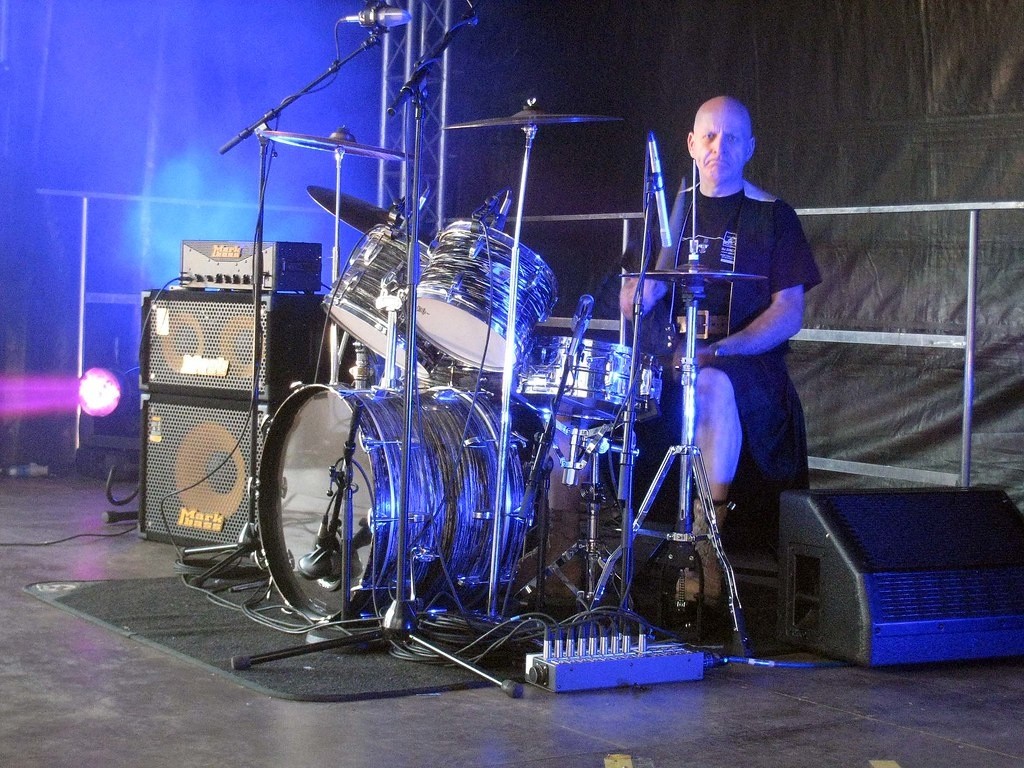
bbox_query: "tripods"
[582,282,756,659]
[173,0,525,701]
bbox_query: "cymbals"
[259,129,407,160]
[305,184,384,236]
[619,268,770,283]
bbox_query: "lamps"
[79,369,142,422]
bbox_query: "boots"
[676,499,736,605]
[503,513,585,604]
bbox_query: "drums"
[256,380,527,619]
[515,336,667,420]
[327,222,445,379]
[410,218,556,373]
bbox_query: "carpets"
[19,577,524,703]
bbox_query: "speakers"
[773,484,1024,670]
[139,288,334,560]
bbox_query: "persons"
[537,95,825,600]
